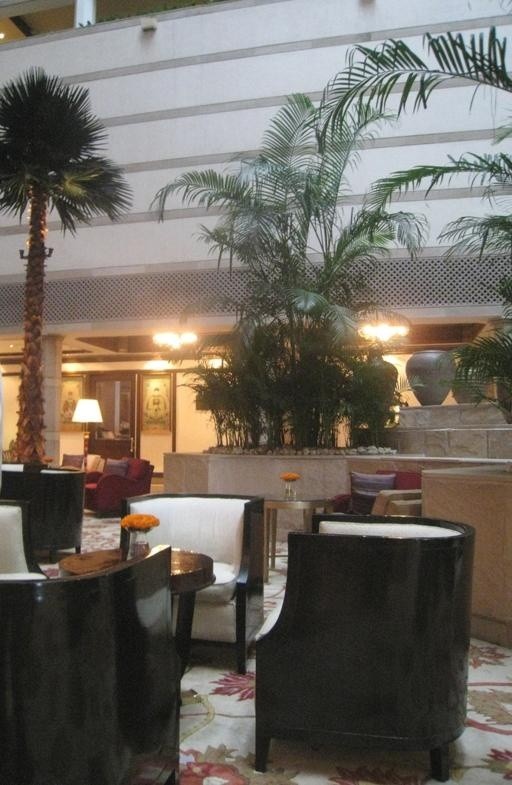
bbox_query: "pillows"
[102,458,129,477]
[349,470,397,516]
[82,453,101,471]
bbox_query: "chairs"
[84,456,155,517]
[0,499,182,785]
[0,468,87,561]
[61,453,83,469]
[331,469,422,518]
[81,456,105,477]
[248,512,476,781]
[0,462,49,473]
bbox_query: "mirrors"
[83,369,139,460]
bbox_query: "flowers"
[279,471,301,482]
[120,513,160,533]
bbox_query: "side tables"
[263,499,328,584]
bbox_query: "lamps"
[71,399,104,471]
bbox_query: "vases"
[496,378,512,423]
[283,481,297,503]
[406,349,458,407]
[125,530,153,560]
[453,365,487,404]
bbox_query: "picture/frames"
[139,372,172,434]
[59,373,87,433]
